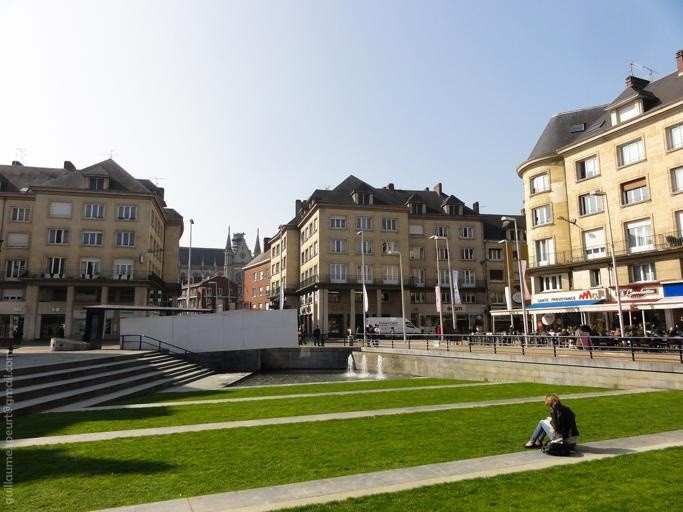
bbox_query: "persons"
[365,324,381,347]
[535,328,570,347]
[313,325,320,346]
[298,325,307,345]
[522,393,580,451]
[57,324,65,338]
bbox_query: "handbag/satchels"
[546,442,569,456]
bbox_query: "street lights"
[436,237,458,332]
[501,217,530,346]
[389,250,407,344]
[499,240,514,327]
[592,191,627,346]
[357,230,366,346]
[186,219,195,315]
[429,235,444,342]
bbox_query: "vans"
[366,317,423,334]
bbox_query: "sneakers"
[525,443,542,448]
